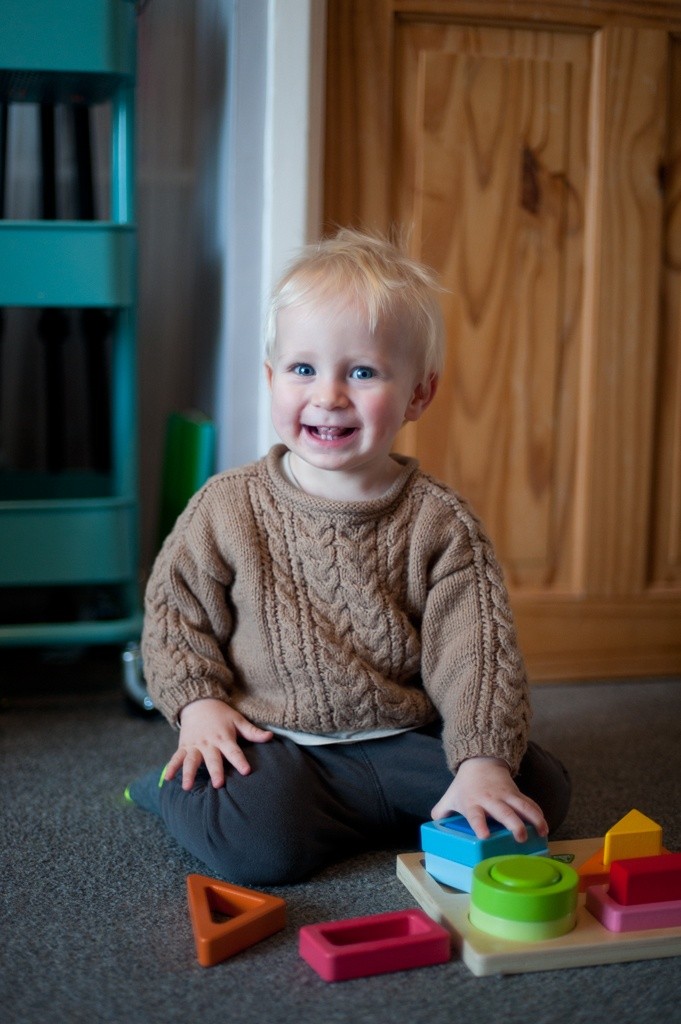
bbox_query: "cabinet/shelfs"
[1,0,162,715]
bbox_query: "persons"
[123,228,567,888]
